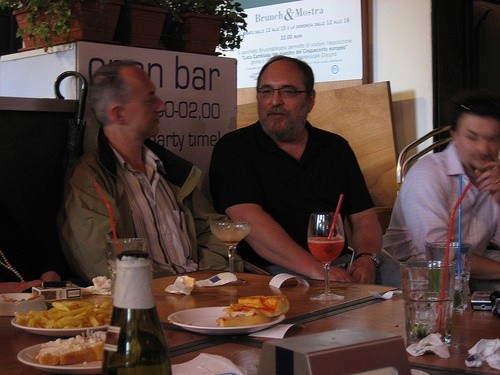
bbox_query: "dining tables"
[1,270,500,375]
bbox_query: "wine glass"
[209,219,253,285]
[307,212,346,302]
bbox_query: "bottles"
[100,250,177,375]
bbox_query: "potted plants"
[0,0,248,57]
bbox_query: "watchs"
[355,253,381,265]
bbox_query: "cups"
[105,237,145,295]
[399,260,457,351]
[423,239,478,312]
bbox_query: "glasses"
[255,85,313,98]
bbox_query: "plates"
[167,305,286,335]
[18,340,104,375]
[10,316,110,337]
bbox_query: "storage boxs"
[30,285,82,301]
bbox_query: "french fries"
[14,300,115,328]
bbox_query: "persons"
[382,91,500,292]
[0,201,61,294]
[58,61,243,284]
[209,55,400,286]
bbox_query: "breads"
[38,336,104,366]
[236,296,290,316]
[217,314,271,327]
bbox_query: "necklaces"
[0,250,24,281]
